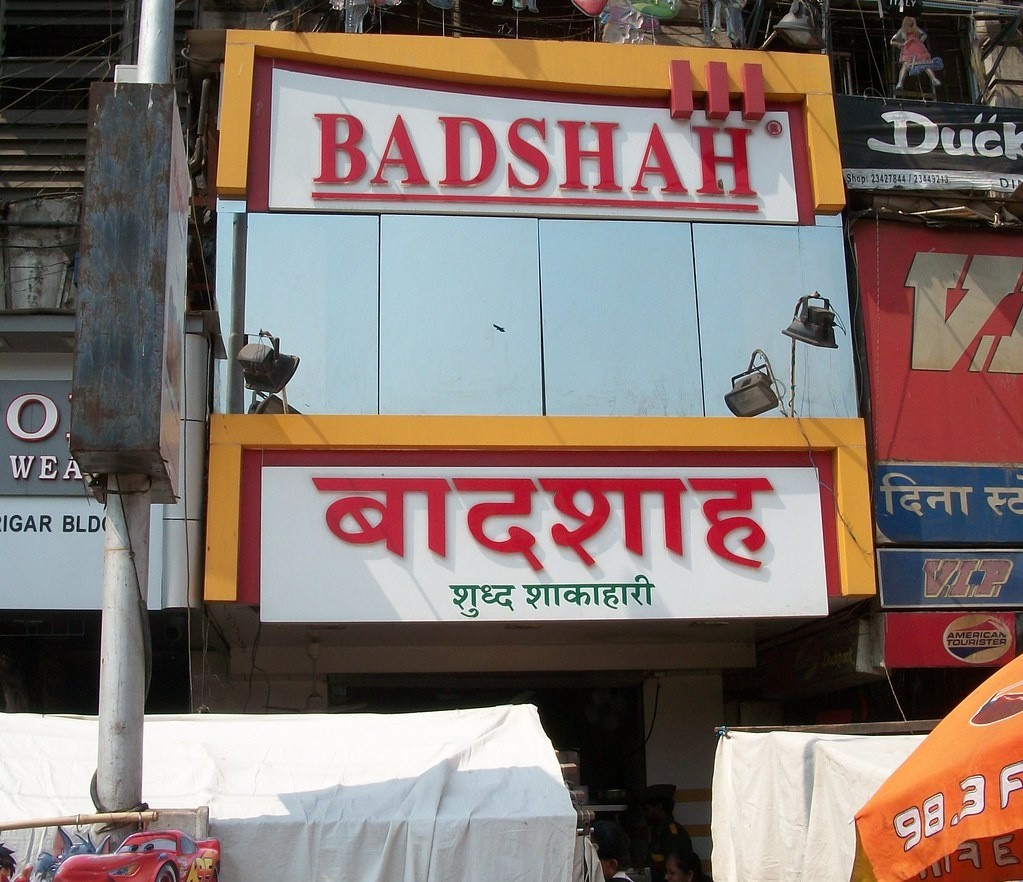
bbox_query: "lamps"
[725,373,780,417]
[782,306,839,348]
[237,344,300,391]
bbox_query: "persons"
[890,17,942,90]
[638,784,695,882]
[664,851,711,882]
[588,821,639,882]
